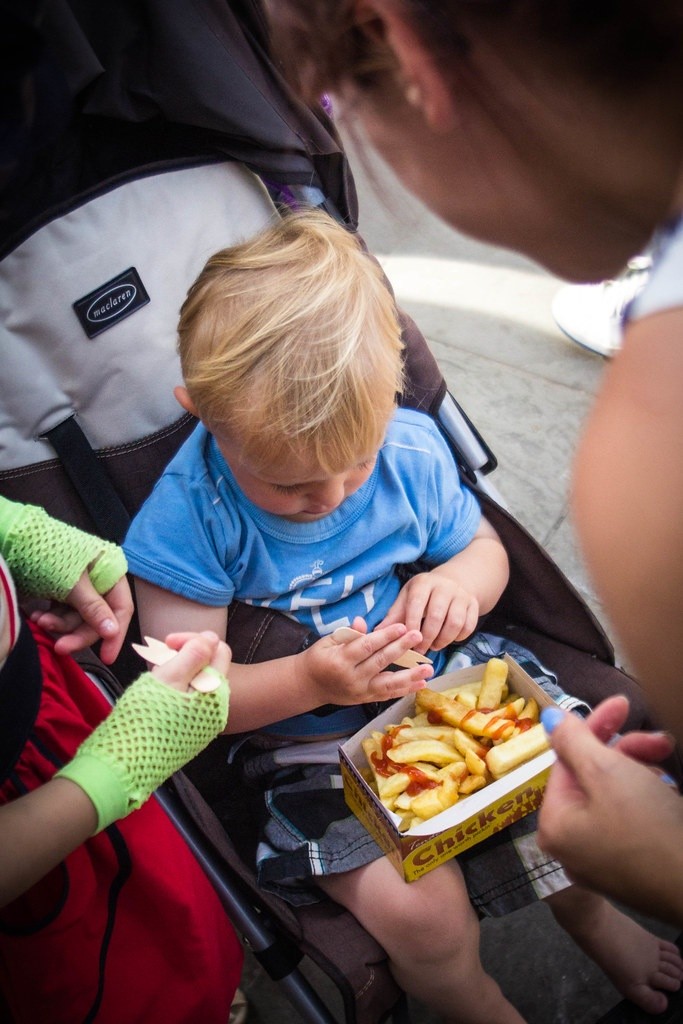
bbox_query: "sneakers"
[552,253,647,357]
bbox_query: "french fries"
[358,656,539,835]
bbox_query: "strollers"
[1,2,682,1024]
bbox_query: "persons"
[124,212,682,1024]
[1,494,243,1024]
[267,0,681,945]
